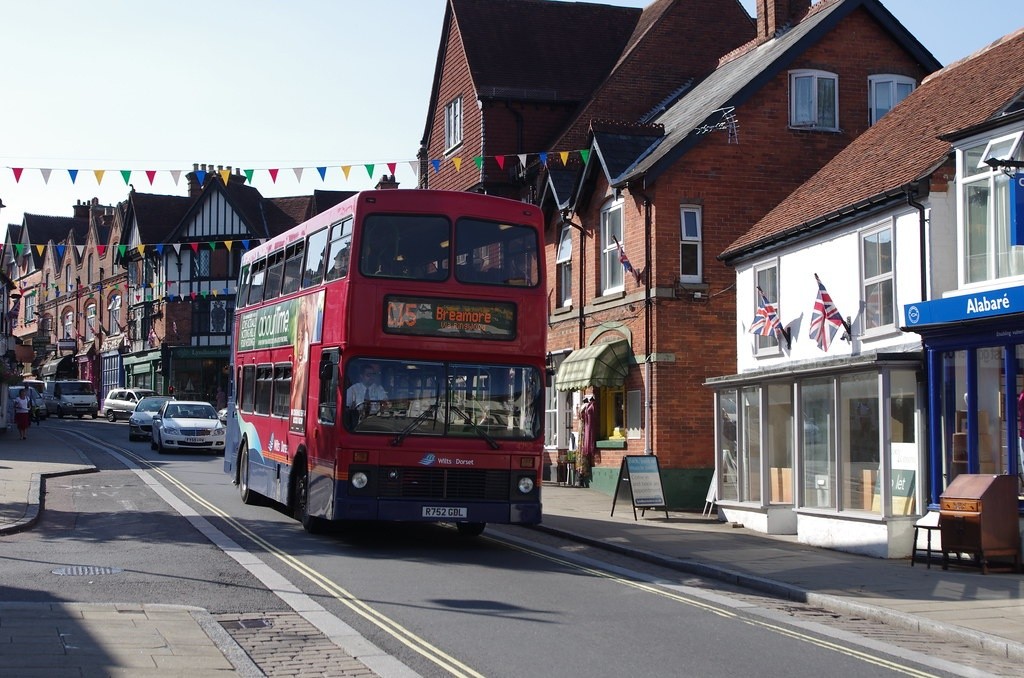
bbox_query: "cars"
[128,395,181,442]
[151,400,227,454]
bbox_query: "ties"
[364,388,371,417]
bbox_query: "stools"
[910,524,949,569]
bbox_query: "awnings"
[555,339,633,392]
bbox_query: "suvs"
[103,386,162,422]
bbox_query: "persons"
[15,383,40,440]
[1017,389,1024,503]
[346,365,388,416]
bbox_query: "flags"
[616,244,641,287]
[748,291,783,344]
[63,313,122,342]
[808,280,843,352]
[148,329,156,347]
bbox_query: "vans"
[8,385,47,420]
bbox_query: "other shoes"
[22,435,27,439]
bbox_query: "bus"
[220,189,554,539]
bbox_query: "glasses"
[362,373,377,378]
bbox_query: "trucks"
[46,379,99,419]
